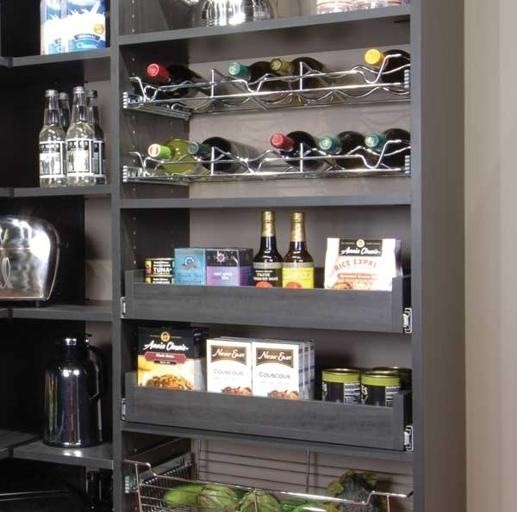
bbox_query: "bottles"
[363,49,411,84]
[39,86,67,183]
[58,91,72,130]
[270,56,334,87]
[253,211,314,291]
[363,127,410,168]
[269,129,322,171]
[86,88,108,184]
[187,135,262,174]
[44,336,102,448]
[147,61,213,114]
[318,131,366,170]
[63,86,101,184]
[148,137,204,176]
[227,59,289,91]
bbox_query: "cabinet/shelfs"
[0,0,467,512]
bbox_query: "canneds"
[361,370,401,406]
[322,368,361,405]
